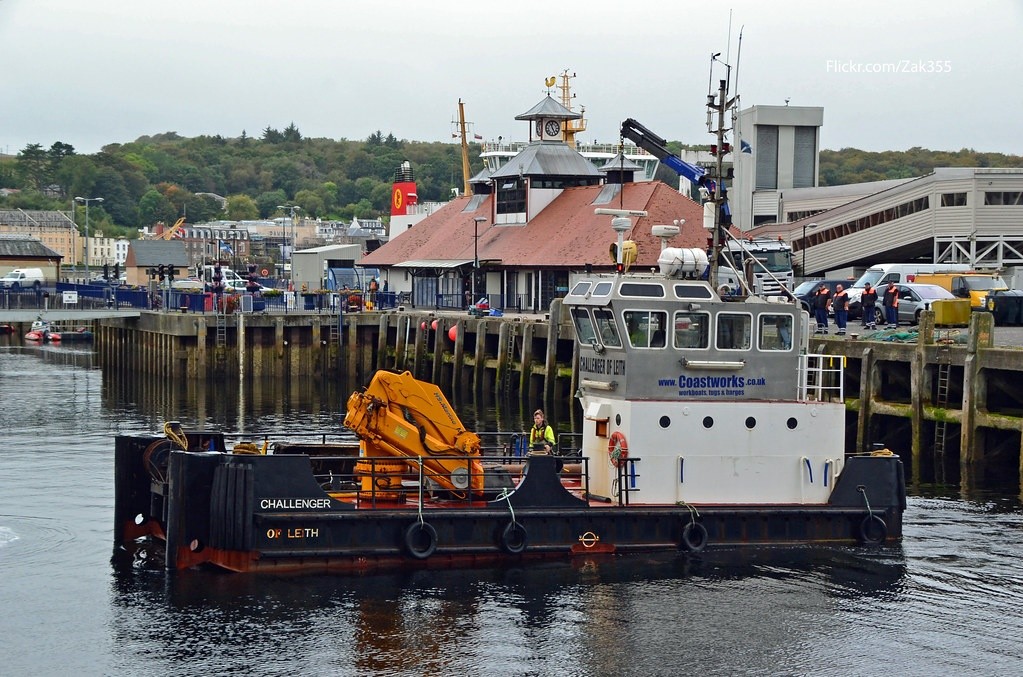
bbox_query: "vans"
[829,263,973,321]
[791,279,856,317]
[914,269,1010,314]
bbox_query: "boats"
[0,324,16,335]
[112,9,908,571]
[24,321,62,342]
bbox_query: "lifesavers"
[608,431,628,468]
[402,521,438,561]
[858,514,887,544]
[499,520,529,555]
[680,521,708,553]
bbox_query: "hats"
[818,282,824,287]
[864,282,870,285]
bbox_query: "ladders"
[216,315,227,346]
[935,365,951,451]
[419,319,430,381]
[807,348,815,394]
[504,322,516,399]
[329,315,338,345]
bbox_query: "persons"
[368,274,378,293]
[814,281,831,334]
[465,281,471,308]
[861,282,877,330]
[627,318,647,347]
[834,283,848,335]
[883,280,900,330]
[383,280,388,291]
[528,410,555,455]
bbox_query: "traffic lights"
[114,262,119,280]
[102,265,109,280]
[158,264,165,281]
[167,264,175,281]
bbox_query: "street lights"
[75,197,104,286]
[209,239,221,298]
[472,217,487,306]
[229,233,236,289]
[801,223,817,276]
[277,205,301,282]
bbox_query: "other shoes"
[887,323,896,329]
[824,331,828,334]
[872,325,877,330]
[864,326,870,329]
[815,329,822,334]
[835,332,846,335]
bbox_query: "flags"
[741,140,751,154]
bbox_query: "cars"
[221,279,274,291]
[863,282,955,326]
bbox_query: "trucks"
[0,268,45,293]
[714,238,796,304]
